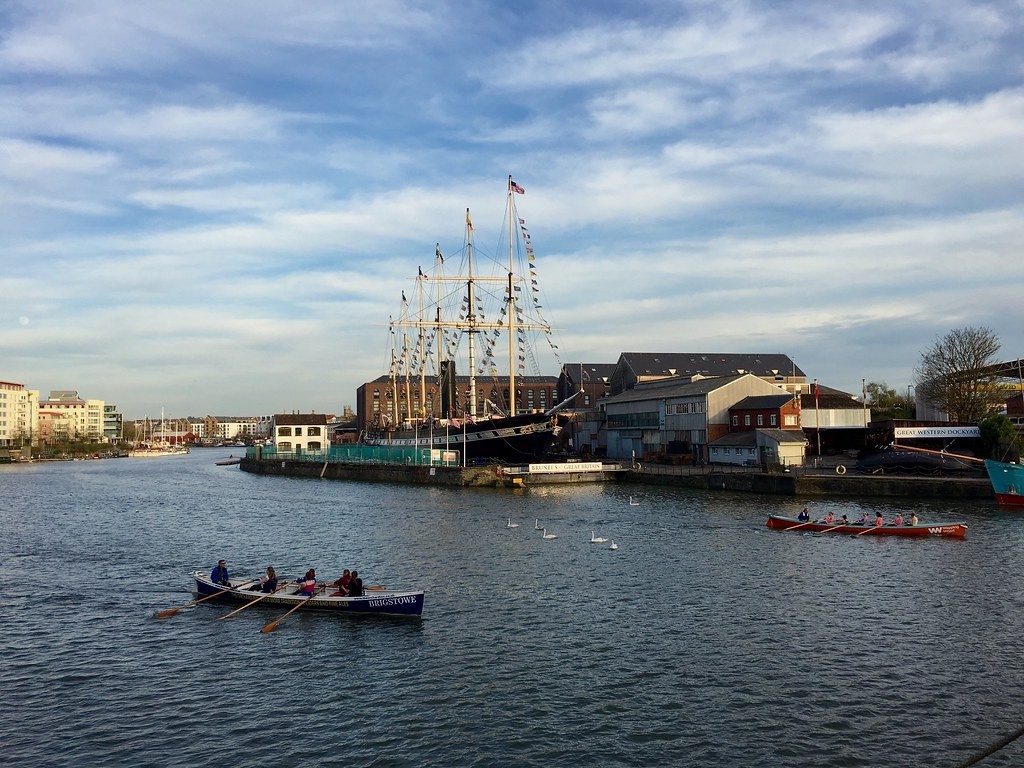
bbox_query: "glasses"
[220,562,225,564]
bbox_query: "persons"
[341,571,362,597]
[326,569,353,597]
[248,567,278,593]
[291,568,316,596]
[905,513,918,526]
[870,511,883,527]
[839,514,849,525]
[798,508,809,522]
[887,511,903,526]
[824,512,834,524]
[857,514,869,525]
[210,560,231,587]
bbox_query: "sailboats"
[126,407,190,458]
[360,171,586,468]
[888,357,1024,507]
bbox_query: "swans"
[543,528,558,539]
[591,531,608,542]
[630,496,640,505]
[610,540,618,550]
[507,518,520,528]
[535,519,545,530]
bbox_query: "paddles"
[155,577,262,618]
[261,586,326,633]
[821,524,846,533]
[784,520,818,531]
[218,581,294,620]
[290,582,331,586]
[362,585,383,590]
[858,526,880,535]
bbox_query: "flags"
[403,414,477,429]
[402,293,407,301]
[436,246,444,264]
[518,215,564,367]
[466,213,474,230]
[475,287,525,388]
[384,296,469,415]
[511,180,525,194]
[419,269,423,276]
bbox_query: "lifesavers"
[836,465,846,475]
[633,462,641,469]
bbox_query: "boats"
[764,511,970,539]
[187,569,426,618]
